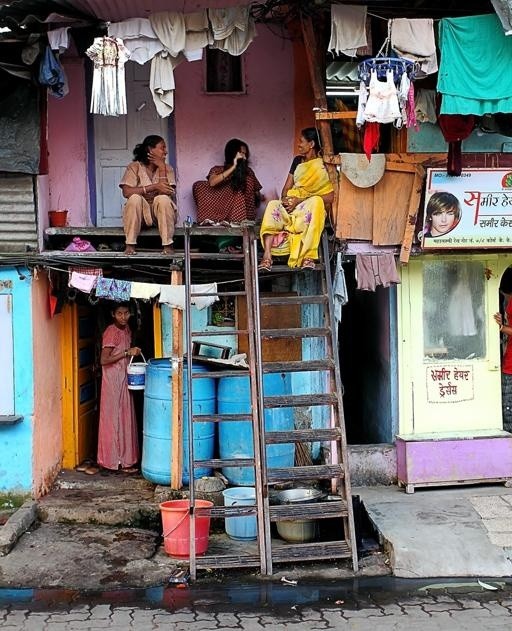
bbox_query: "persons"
[119,134,179,253]
[492,284,512,435]
[97,301,141,472]
[424,191,461,238]
[191,138,263,228]
[259,126,336,273]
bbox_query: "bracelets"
[124,348,129,357]
[142,185,146,195]
[499,324,504,332]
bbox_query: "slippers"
[76,459,99,475]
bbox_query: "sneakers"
[257,258,273,274]
[300,259,316,271]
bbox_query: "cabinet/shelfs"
[395,429,512,494]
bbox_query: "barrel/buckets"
[127,352,149,390]
[158,500,214,560]
[217,372,296,486]
[222,486,258,541]
[276,488,323,542]
[141,358,216,486]
[48,209,68,227]
